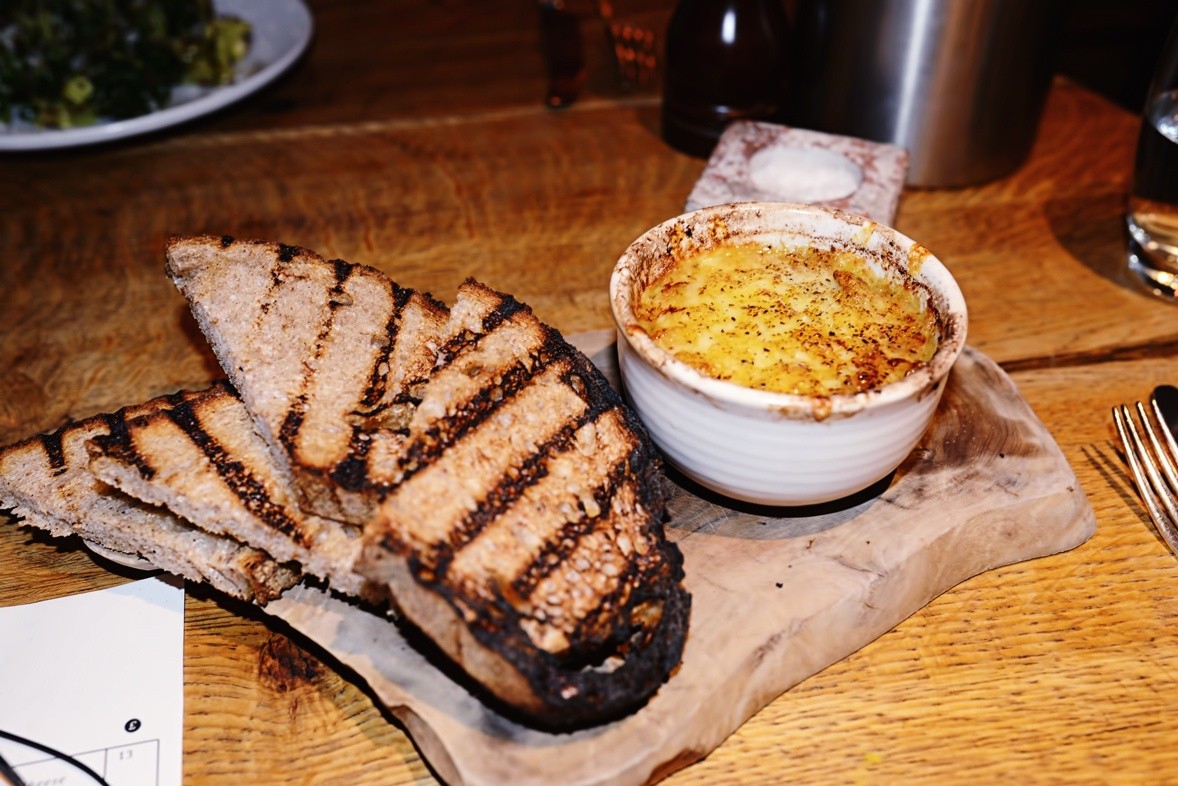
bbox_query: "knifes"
[534,0,585,107]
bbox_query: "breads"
[0,235,692,735]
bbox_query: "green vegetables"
[0,0,253,130]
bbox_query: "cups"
[818,1,1065,188]
[1121,20,1178,303]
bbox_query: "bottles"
[661,0,828,160]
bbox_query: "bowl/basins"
[608,199,969,516]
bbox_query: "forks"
[592,1,657,92]
[1112,398,1178,560]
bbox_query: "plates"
[0,1,314,152]
